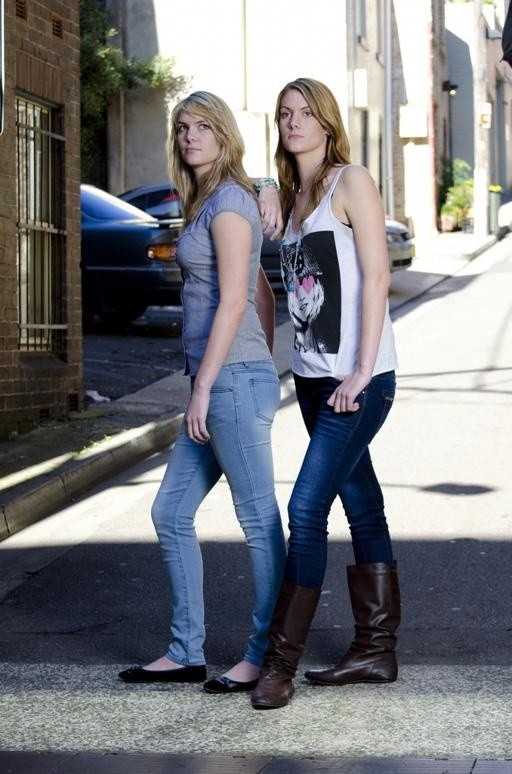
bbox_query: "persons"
[114,88,295,694]
[234,69,402,710]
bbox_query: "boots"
[249,568,324,709]
[303,559,404,686]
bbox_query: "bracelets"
[252,176,280,193]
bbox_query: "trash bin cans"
[488,185,504,234]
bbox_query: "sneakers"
[203,673,262,693]
[118,660,211,684]
